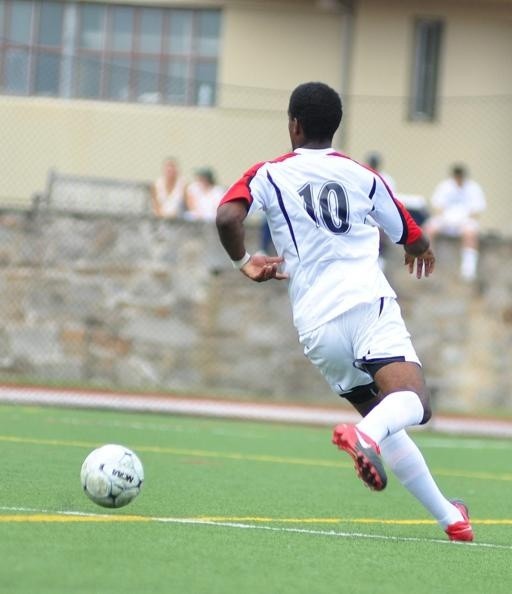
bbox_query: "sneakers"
[446,498,475,543]
[332,422,387,492]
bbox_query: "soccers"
[81,445,144,507]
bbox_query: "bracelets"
[230,249,250,269]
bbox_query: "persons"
[418,159,488,287]
[145,154,187,219]
[362,150,395,196]
[181,165,227,220]
[214,81,474,542]
